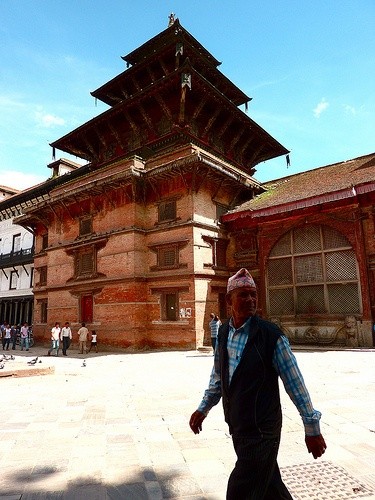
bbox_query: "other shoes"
[26,349,29,351]
[56,355,60,357]
[48,351,50,355]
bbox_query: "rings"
[323,447,325,451]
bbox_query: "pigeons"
[0,353,15,369]
[27,356,43,365]
[83,360,87,366]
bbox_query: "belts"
[63,337,69,338]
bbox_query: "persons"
[209,313,222,356]
[48,322,98,357]
[0,321,29,351]
[189,267,327,500]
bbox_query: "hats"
[226,268,257,294]
